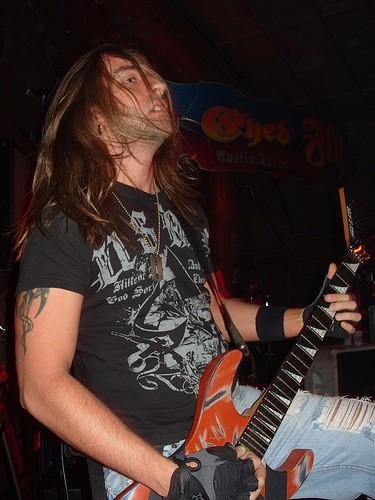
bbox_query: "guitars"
[111,185,374,499]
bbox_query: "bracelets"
[255,302,287,344]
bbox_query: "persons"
[12,43,374,500]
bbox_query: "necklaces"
[108,176,164,282]
